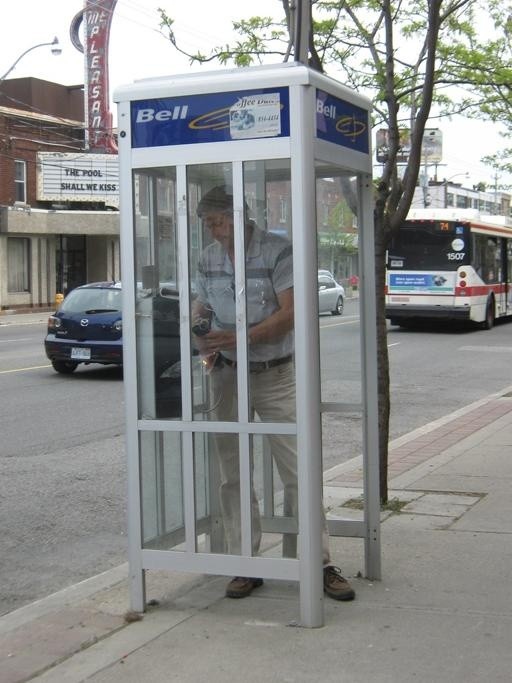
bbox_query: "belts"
[216,352,292,373]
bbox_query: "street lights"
[422,171,471,206]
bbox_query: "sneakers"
[225,577,263,598]
[324,567,355,601]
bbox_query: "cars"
[318,269,346,317]
[44,273,197,374]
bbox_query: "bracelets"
[248,335,252,344]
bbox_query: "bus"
[383,207,512,331]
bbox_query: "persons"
[188,184,356,601]
